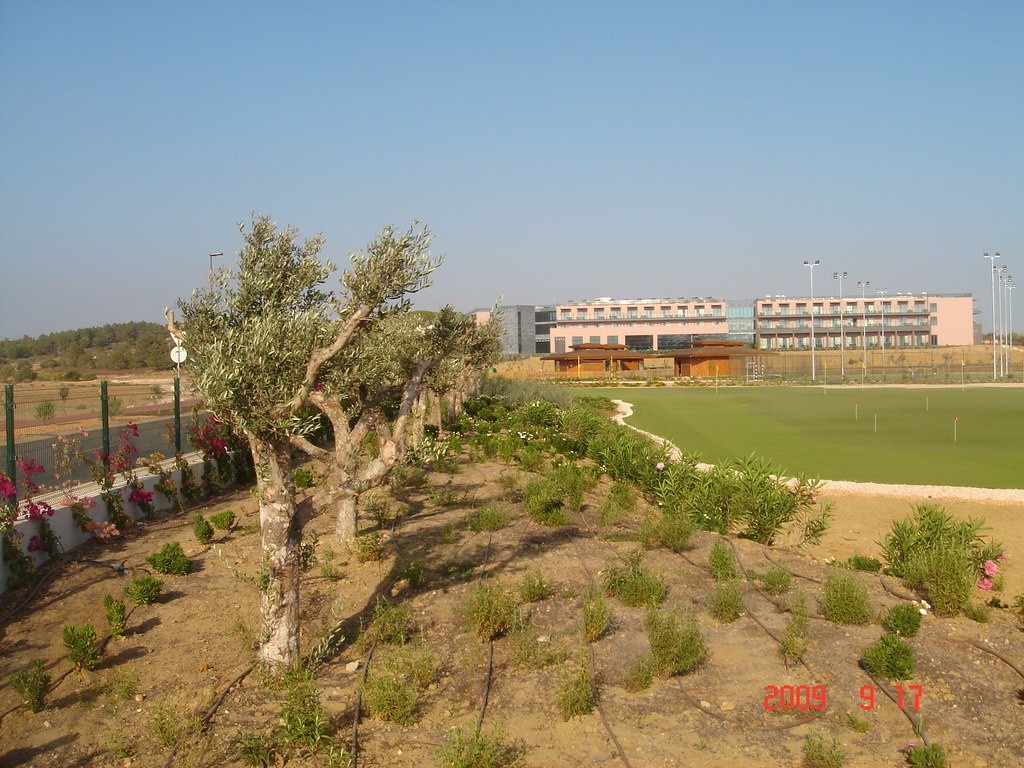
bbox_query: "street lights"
[833,271,848,374]
[992,264,1006,376]
[983,251,1001,379]
[856,280,870,374]
[1006,282,1017,373]
[209,251,223,290]
[1000,275,1009,378]
[876,288,887,372]
[804,259,820,381]
